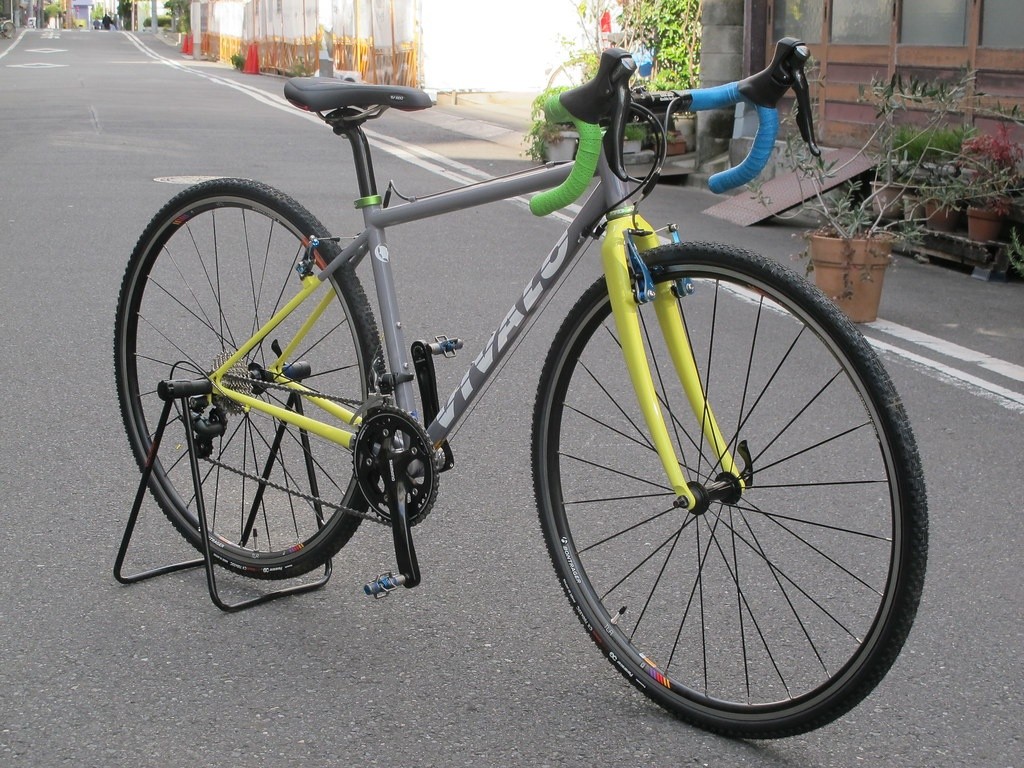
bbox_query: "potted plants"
[869,125,1024,242]
[519,0,703,164]
[743,53,1024,322]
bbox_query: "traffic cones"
[241,42,259,74]
[186,35,193,56]
[178,34,188,54]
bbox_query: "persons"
[102,14,114,30]
[93,19,101,30]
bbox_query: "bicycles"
[0,17,16,40]
[109,33,933,742]
